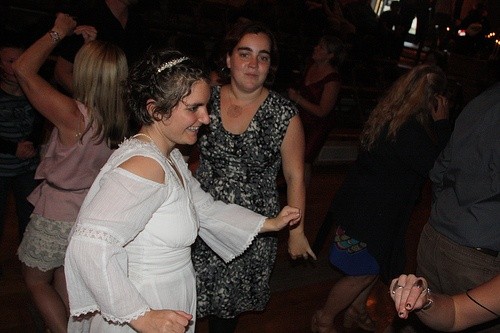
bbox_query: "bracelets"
[423,288,433,309]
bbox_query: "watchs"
[49,28,60,42]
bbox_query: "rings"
[412,280,424,290]
[391,280,404,294]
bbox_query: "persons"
[388,270,500,333]
[416,79,500,295]
[311,65,455,333]
[63,51,302,333]
[298,0,500,91]
[282,34,346,197]
[0,39,45,248]
[13,14,130,332]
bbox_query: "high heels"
[344,307,378,331]
[311,312,339,333]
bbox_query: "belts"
[466,245,499,258]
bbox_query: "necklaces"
[132,133,175,168]
